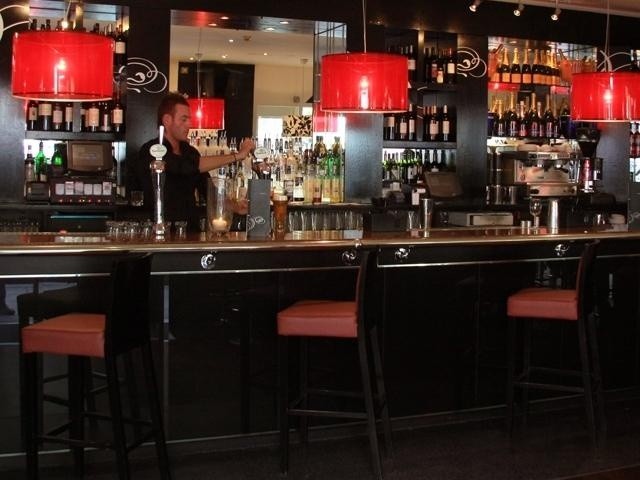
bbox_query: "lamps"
[318,1,412,116]
[568,1,639,124]
[9,0,116,104]
[184,26,224,131]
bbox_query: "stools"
[14,252,170,480]
[267,248,399,480]
[501,239,608,458]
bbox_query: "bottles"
[24,19,125,205]
[189,130,364,241]
[382,45,456,185]
[0,221,40,233]
[487,48,640,158]
[579,157,593,190]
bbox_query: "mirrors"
[170,10,346,203]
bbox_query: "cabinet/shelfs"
[19,17,129,206]
[487,35,597,207]
[345,24,457,209]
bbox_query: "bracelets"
[233,152,238,163]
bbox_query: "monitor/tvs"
[423,171,464,199]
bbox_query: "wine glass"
[528,197,544,232]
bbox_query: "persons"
[136,93,256,231]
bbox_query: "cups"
[131,190,144,206]
[104,218,205,245]
